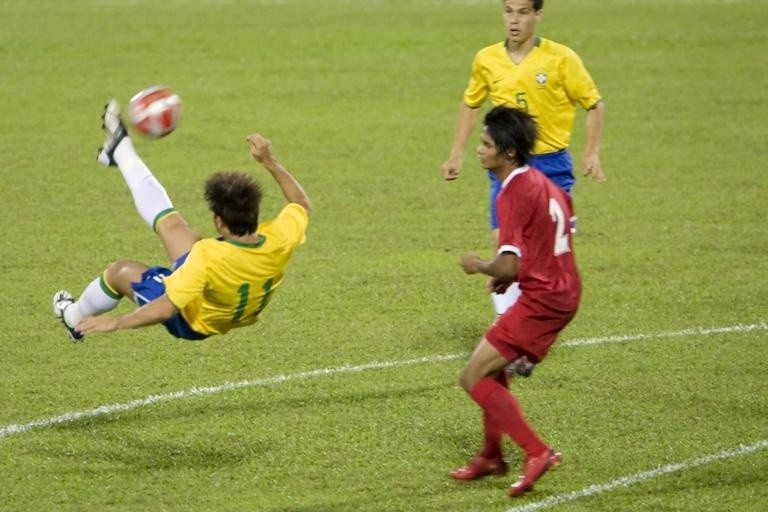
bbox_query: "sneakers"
[517,356,534,377]
[448,457,508,481]
[53,290,86,342]
[96,99,127,167]
[509,447,560,497]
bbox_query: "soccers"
[128,85,181,139]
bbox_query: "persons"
[448,103,581,499]
[437,0,605,379]
[53,98,312,346]
